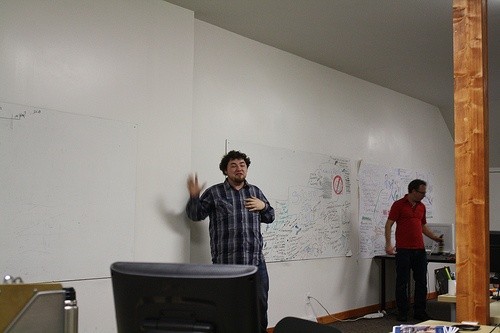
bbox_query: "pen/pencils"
[444,265,452,280]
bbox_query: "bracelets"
[261,201,266,211]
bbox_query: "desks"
[375,255,455,311]
[438,293,457,321]
[415,319,500,333]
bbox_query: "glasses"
[416,190,425,195]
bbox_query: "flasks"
[63,299,78,333]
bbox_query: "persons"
[185,151,275,333]
[384,179,444,321]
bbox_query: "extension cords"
[363,312,383,319]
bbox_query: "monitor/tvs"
[490,230,500,284]
[422,223,454,254]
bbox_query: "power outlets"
[306,294,310,304]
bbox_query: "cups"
[448,280,456,296]
